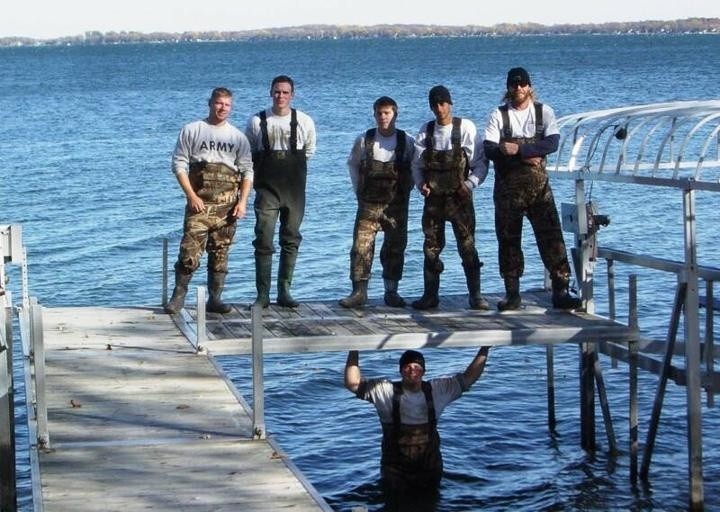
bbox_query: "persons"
[244,75,316,310]
[339,96,418,308]
[164,88,254,314]
[410,85,489,309]
[482,66,582,311]
[344,348,490,487]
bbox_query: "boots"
[497,276,523,311]
[338,279,369,309]
[252,261,272,311]
[277,261,300,308]
[549,278,584,309]
[164,273,194,314]
[410,266,446,310]
[207,272,234,313]
[383,279,407,308]
[461,265,491,309]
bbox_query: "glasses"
[508,82,530,89]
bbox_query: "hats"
[428,85,453,106]
[506,67,532,86]
[399,350,426,368]
